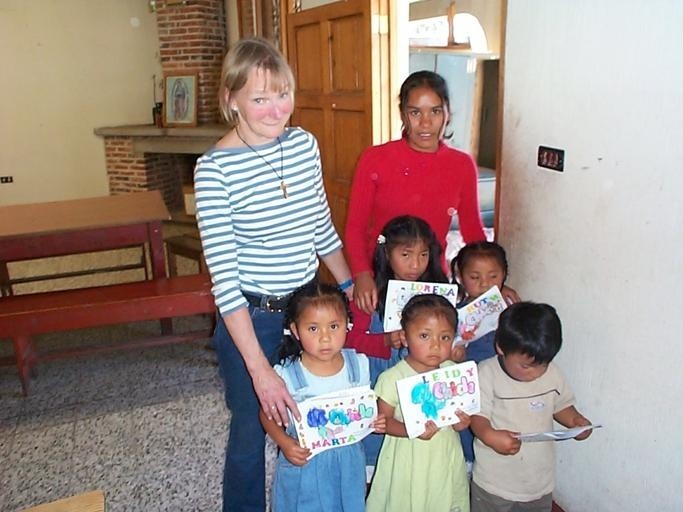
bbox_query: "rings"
[270,405,275,411]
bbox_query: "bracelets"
[338,279,352,291]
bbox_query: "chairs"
[163,227,218,329]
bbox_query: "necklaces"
[232,124,288,200]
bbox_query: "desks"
[0,189,175,337]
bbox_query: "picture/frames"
[162,69,199,128]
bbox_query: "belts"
[242,272,321,313]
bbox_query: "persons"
[250,279,389,512]
[341,70,524,319]
[360,291,475,512]
[334,214,454,466]
[191,34,356,512]
[463,299,594,512]
[447,240,517,467]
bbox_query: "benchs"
[0,273,218,398]
